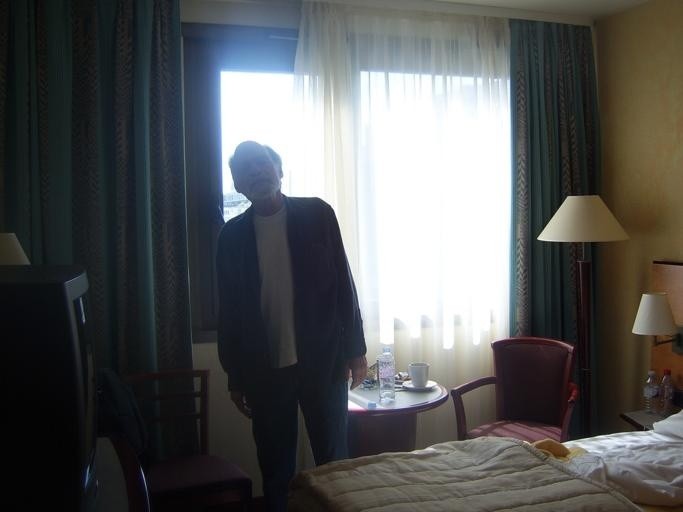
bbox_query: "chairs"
[450,335,579,445]
[118,369,251,511]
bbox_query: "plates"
[402,380,438,391]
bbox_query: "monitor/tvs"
[0,261,98,511]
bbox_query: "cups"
[409,362,430,387]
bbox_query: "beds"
[289,407,682,511]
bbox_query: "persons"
[214,140,369,512]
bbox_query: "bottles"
[378,348,396,401]
[643,370,660,414]
[659,369,674,414]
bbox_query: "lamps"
[536,195,630,437]
[631,291,683,356]
